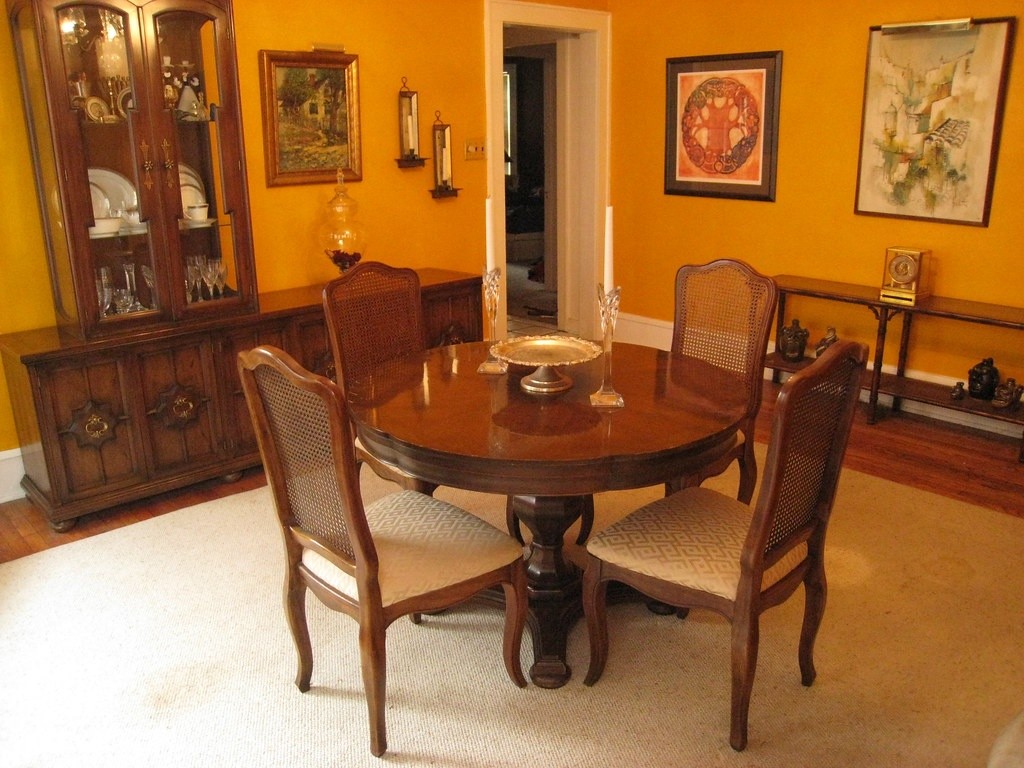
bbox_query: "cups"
[110,208,139,228]
[99,113,120,122]
[183,204,208,219]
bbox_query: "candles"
[485,196,495,273]
[443,147,447,181]
[407,114,413,149]
[604,205,614,295]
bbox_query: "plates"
[85,96,110,120]
[177,162,207,219]
[88,227,120,238]
[117,87,136,118]
[489,335,604,366]
[178,219,217,228]
[86,166,138,223]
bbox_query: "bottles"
[815,325,840,360]
[781,320,807,361]
[953,382,966,399]
[321,167,370,272]
[966,357,1000,400]
[993,376,1023,410]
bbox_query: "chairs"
[235,343,529,758]
[660,255,779,506]
[577,340,871,754]
[320,260,527,623]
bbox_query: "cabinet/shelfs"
[753,273,1024,426]
[2,0,488,539]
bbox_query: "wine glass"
[183,255,228,305]
[95,265,132,318]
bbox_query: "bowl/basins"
[94,218,120,232]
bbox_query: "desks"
[349,341,753,692]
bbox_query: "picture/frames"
[258,49,362,189]
[664,49,776,205]
[853,16,1017,226]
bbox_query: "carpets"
[0,439,1024,766]
